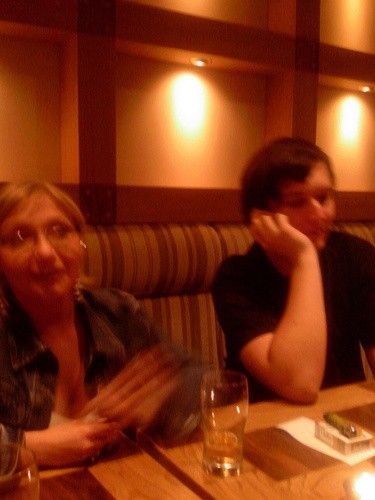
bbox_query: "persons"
[208,137,375,406]
[1,180,203,471]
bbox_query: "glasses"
[0,220,76,247]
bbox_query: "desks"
[0,376,375,500]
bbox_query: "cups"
[0,442,40,500]
[201,370,249,478]
